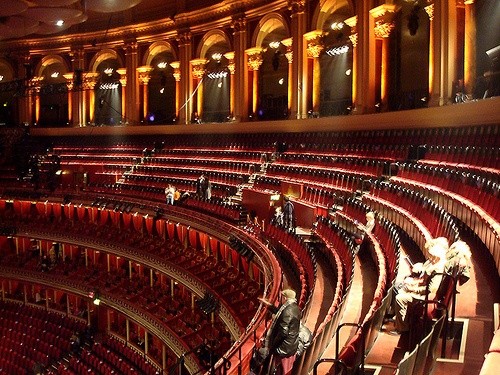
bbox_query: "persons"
[171,186,181,205]
[388,245,448,332]
[165,185,174,205]
[41,254,50,272]
[351,211,376,255]
[385,237,449,318]
[271,205,283,227]
[36,289,43,305]
[283,196,296,231]
[70,331,80,353]
[197,173,208,199]
[296,321,312,360]
[78,305,88,319]
[251,289,300,374]
[197,338,213,364]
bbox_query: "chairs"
[1,138,500,375]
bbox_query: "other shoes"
[381,317,398,333]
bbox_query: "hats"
[280,288,297,299]
